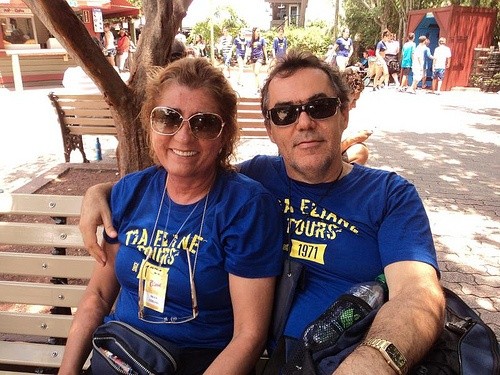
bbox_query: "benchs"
[46,89,271,163]
[0,190,111,375]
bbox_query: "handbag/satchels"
[90,320,183,375]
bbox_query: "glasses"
[265,96,343,128]
[149,106,228,141]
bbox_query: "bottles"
[302,274,386,351]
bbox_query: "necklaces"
[144,167,219,267]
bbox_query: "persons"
[247,26,269,92]
[422,39,434,88]
[406,36,428,93]
[174,26,247,86]
[428,37,451,95]
[62,36,122,139]
[397,32,417,92]
[328,28,354,73]
[2,19,28,44]
[92,22,143,73]
[272,28,288,67]
[325,45,337,64]
[57,57,286,375]
[338,68,372,166]
[354,30,400,92]
[78,51,450,375]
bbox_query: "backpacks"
[277,274,500,375]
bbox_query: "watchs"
[358,337,409,375]
